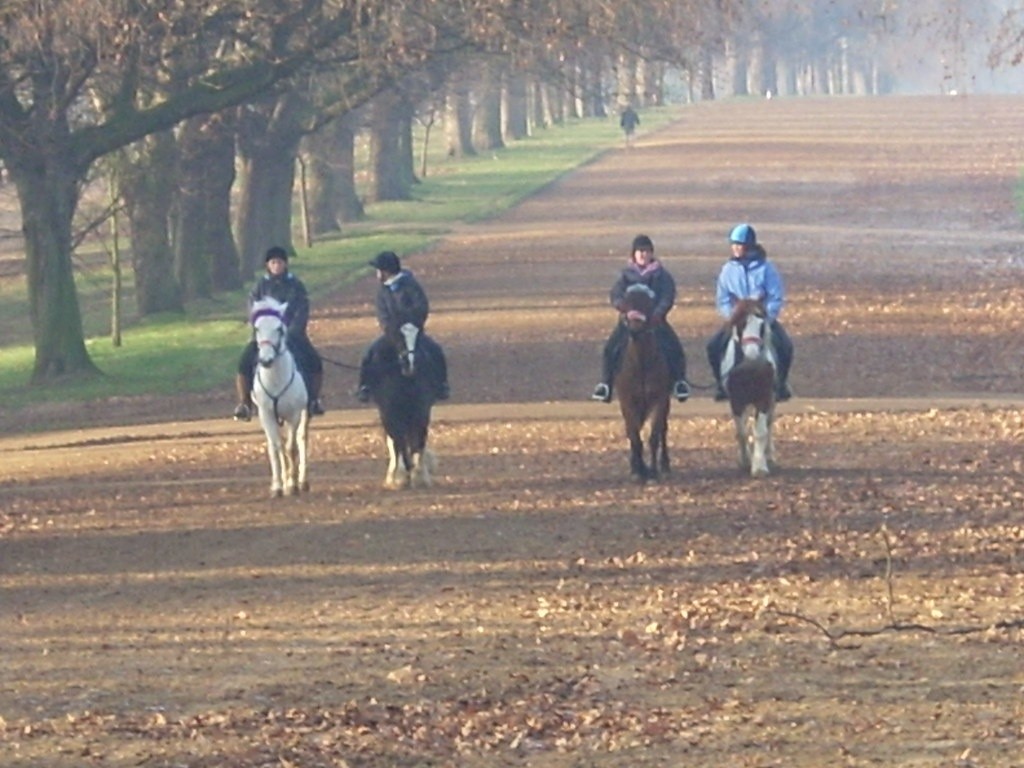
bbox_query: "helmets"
[369,252,400,274]
[728,224,756,246]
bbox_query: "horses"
[246,300,310,499]
[612,273,676,478]
[374,312,432,491]
[724,291,779,478]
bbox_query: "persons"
[233,247,324,422]
[707,225,794,399]
[594,235,689,402]
[621,107,640,150]
[357,252,449,402]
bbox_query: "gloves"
[649,313,663,326]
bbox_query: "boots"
[233,373,254,416]
[308,370,324,414]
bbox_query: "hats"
[266,247,287,263]
[633,235,652,249]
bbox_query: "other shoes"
[714,389,728,401]
[677,384,687,402]
[439,384,450,399]
[359,384,369,402]
[596,387,612,403]
[777,387,791,399]
